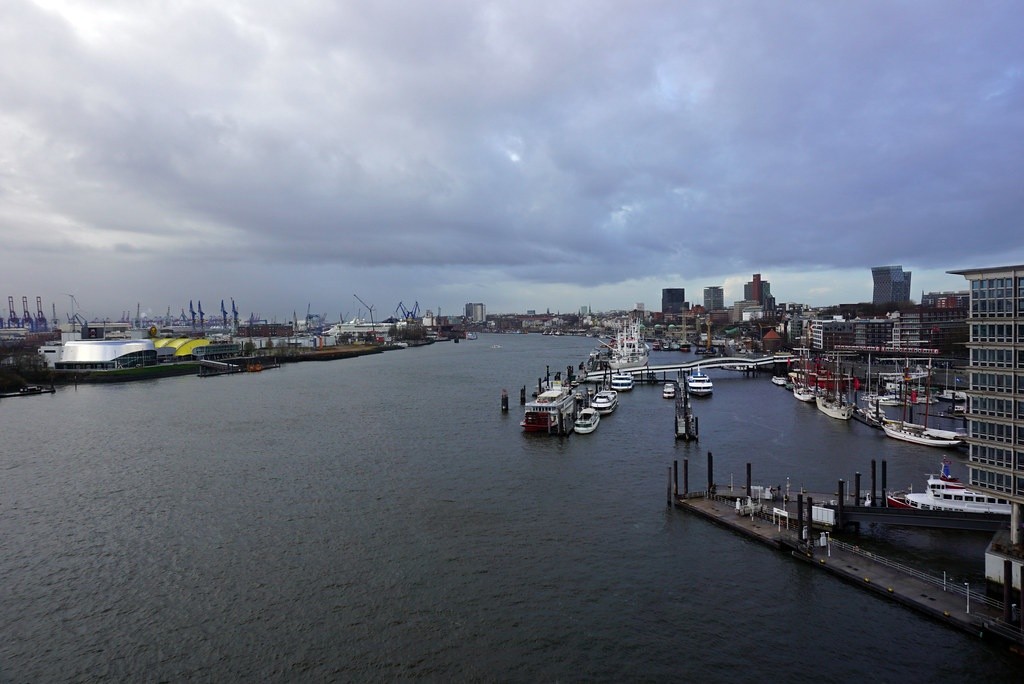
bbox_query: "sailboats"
[772,357,962,448]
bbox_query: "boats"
[685,362,713,396]
[652,342,690,352]
[607,318,649,369]
[587,388,593,396]
[19,386,54,394]
[542,329,563,336]
[663,384,676,398]
[573,407,600,435]
[591,387,618,415]
[492,345,502,349]
[885,455,1011,515]
[519,386,576,432]
[575,392,582,399]
[610,373,636,393]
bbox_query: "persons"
[143,326,159,339]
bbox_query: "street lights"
[964,583,970,613]
[826,532,830,557]
